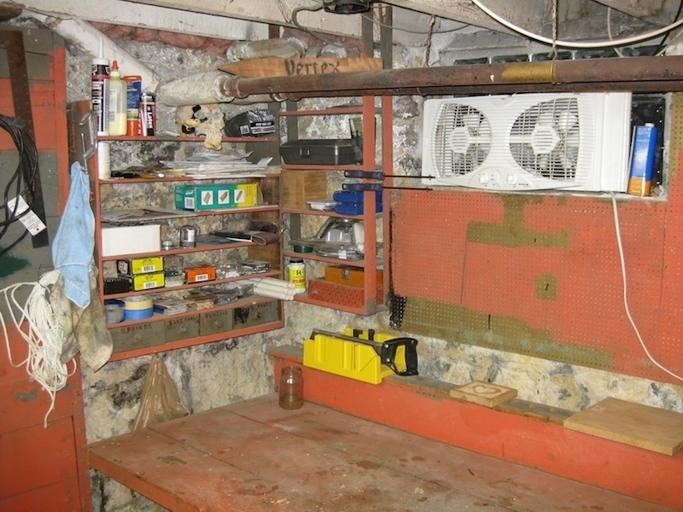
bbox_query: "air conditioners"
[423,93,633,193]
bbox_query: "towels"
[52,161,94,307]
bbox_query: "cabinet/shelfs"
[388,89,683,385]
[0,31,95,512]
[68,96,283,364]
[276,89,387,316]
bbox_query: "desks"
[88,394,679,512]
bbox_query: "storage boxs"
[175,182,265,211]
[118,271,165,291]
[308,277,384,310]
[281,139,364,164]
[266,344,683,510]
[116,256,165,275]
[326,266,383,288]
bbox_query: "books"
[99,206,199,225]
[155,151,278,178]
